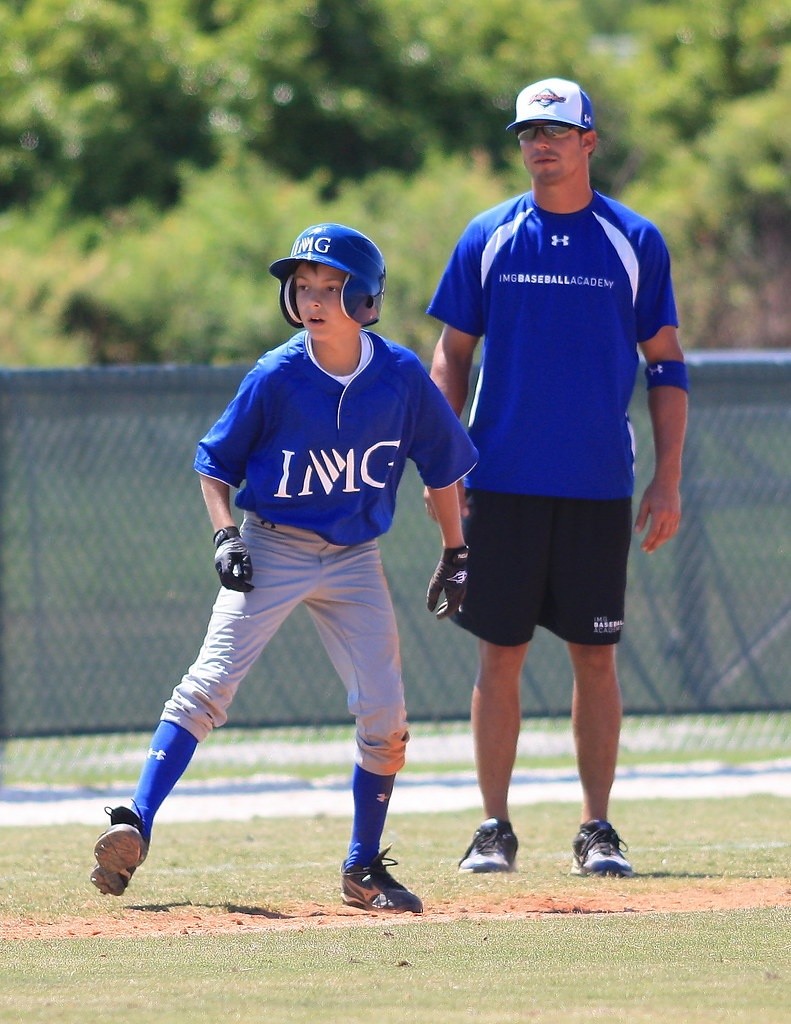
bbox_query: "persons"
[425,78,692,878]
[91,224,479,914]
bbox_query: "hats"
[505,78,594,132]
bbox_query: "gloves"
[426,545,470,620]
[213,525,255,592]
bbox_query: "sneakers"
[571,819,633,877]
[341,842,424,914]
[90,799,149,896]
[459,817,518,873]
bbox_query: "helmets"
[268,223,386,328]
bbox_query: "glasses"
[515,122,580,142]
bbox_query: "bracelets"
[645,360,690,392]
